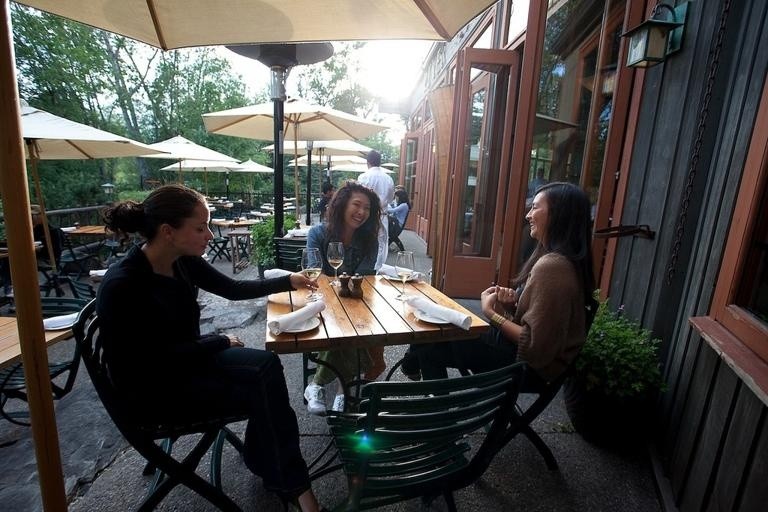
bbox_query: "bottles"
[351,272,363,298]
[294,221,301,229]
[338,271,351,297]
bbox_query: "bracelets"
[489,312,509,331]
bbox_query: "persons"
[321,181,335,210]
[299,181,383,417]
[391,193,409,238]
[394,185,404,193]
[21,202,63,266]
[401,179,596,398]
[355,149,394,275]
[96,185,320,512]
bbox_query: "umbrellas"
[14,97,169,292]
[126,95,400,176]
[0,0,495,512]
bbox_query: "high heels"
[282,487,327,512]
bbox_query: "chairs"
[457,300,601,491]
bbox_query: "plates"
[413,305,456,324]
[381,272,418,281]
[279,316,321,334]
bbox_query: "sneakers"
[332,394,344,411]
[303,384,328,416]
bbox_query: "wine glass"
[301,248,324,302]
[326,241,344,286]
[395,251,415,301]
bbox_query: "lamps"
[619,1,688,69]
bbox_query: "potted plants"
[564,288,670,452]
